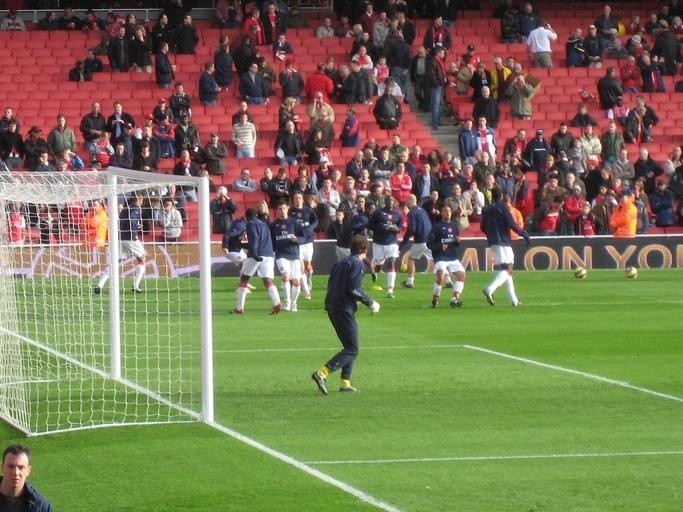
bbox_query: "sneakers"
[232,271,523,314]
[312,371,328,394]
[132,286,142,292]
[339,387,358,392]
[94,286,100,294]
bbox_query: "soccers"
[575,266,587,280]
[625,267,637,279]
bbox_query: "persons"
[2,443,52,512]
[0,0,683,315]
[311,233,380,395]
